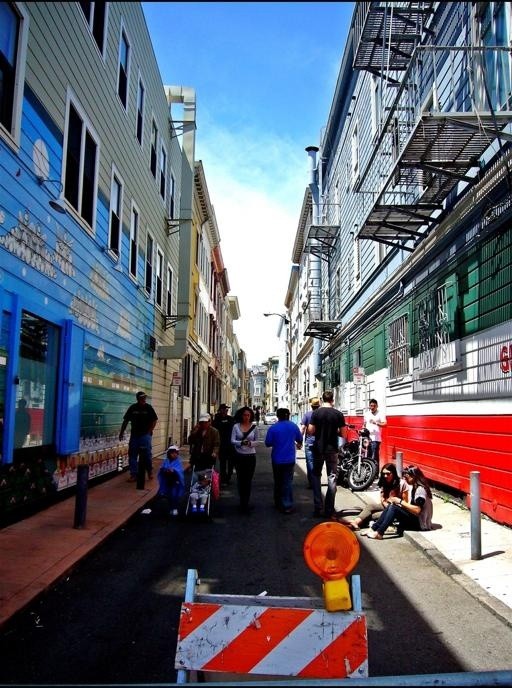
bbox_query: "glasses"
[382,472,391,476]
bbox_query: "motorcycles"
[337,422,378,491]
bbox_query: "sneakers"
[192,507,197,512]
[173,509,178,515]
[148,474,153,480]
[170,510,173,515]
[200,507,205,512]
[129,477,136,482]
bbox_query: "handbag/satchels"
[211,468,220,500]
[229,425,255,464]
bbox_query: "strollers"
[185,461,220,519]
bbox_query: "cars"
[264,412,278,425]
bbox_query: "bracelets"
[400,499,403,504]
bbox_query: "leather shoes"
[360,531,368,535]
[367,532,383,540]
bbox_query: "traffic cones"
[169,567,374,681]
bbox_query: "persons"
[307,391,345,518]
[211,404,236,487]
[360,464,433,540]
[232,406,260,504]
[363,400,386,479]
[255,407,262,428]
[300,399,323,488]
[190,414,220,468]
[346,463,408,530]
[191,474,209,514]
[119,391,158,481]
[266,408,303,513]
[157,445,187,516]
[15,399,33,447]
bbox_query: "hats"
[198,413,211,422]
[311,399,320,407]
[136,391,147,398]
[277,408,289,416]
[218,404,229,412]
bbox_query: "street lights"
[264,311,290,326]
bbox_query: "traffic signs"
[352,367,367,387]
[170,372,183,388]
